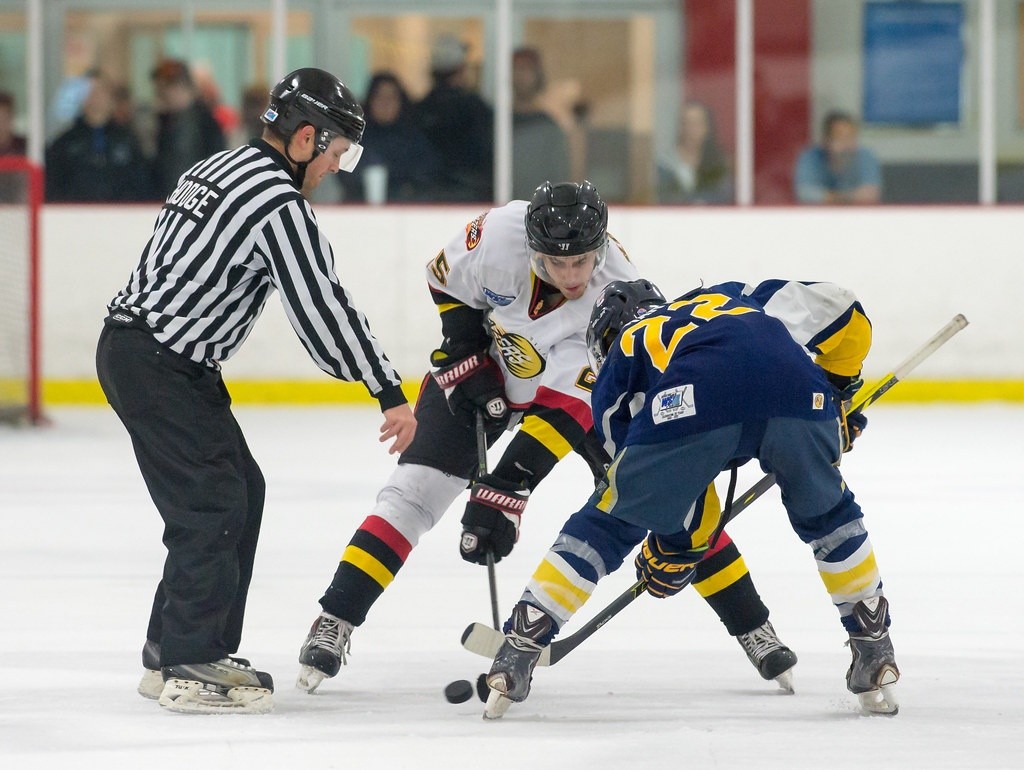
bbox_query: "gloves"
[843,379,868,454]
[431,336,509,432]
[634,529,706,598]
[460,474,531,564]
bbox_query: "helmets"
[585,278,668,360]
[524,178,608,257]
[260,68,366,155]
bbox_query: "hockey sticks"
[461,313,969,669]
[475,405,534,706]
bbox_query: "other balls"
[445,679,474,704]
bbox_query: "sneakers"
[295,607,356,694]
[158,654,275,715]
[844,596,900,715]
[136,637,252,699]
[735,614,797,695]
[480,601,554,722]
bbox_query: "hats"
[150,59,182,87]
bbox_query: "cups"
[362,165,387,204]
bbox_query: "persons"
[656,103,727,207]
[94,68,420,716]
[483,279,899,713]
[0,62,227,203]
[314,34,565,200]
[793,114,881,204]
[300,180,798,693]
